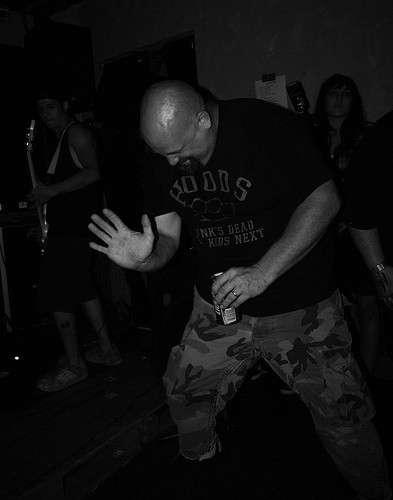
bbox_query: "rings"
[232,290,239,298]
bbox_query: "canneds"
[211,272,243,326]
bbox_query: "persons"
[26,90,123,393]
[341,110,393,376]
[86,80,393,499]
[315,72,382,337]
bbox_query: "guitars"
[24,119,49,256]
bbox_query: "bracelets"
[369,263,388,272]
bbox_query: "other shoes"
[34,363,87,392]
[148,447,218,479]
[82,348,121,364]
